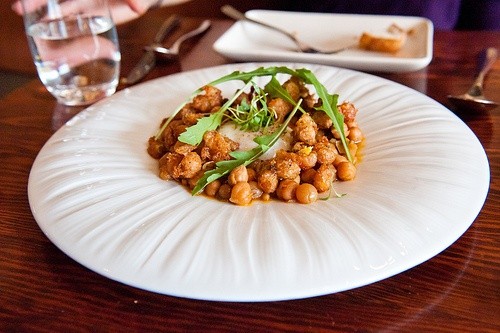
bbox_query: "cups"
[23,0,121,106]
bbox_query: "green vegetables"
[155,66,353,197]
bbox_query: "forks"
[221,5,350,55]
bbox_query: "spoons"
[144,20,211,57]
[447,48,500,110]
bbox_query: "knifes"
[127,14,180,84]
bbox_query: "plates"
[29,63,491,302]
[213,10,434,72]
